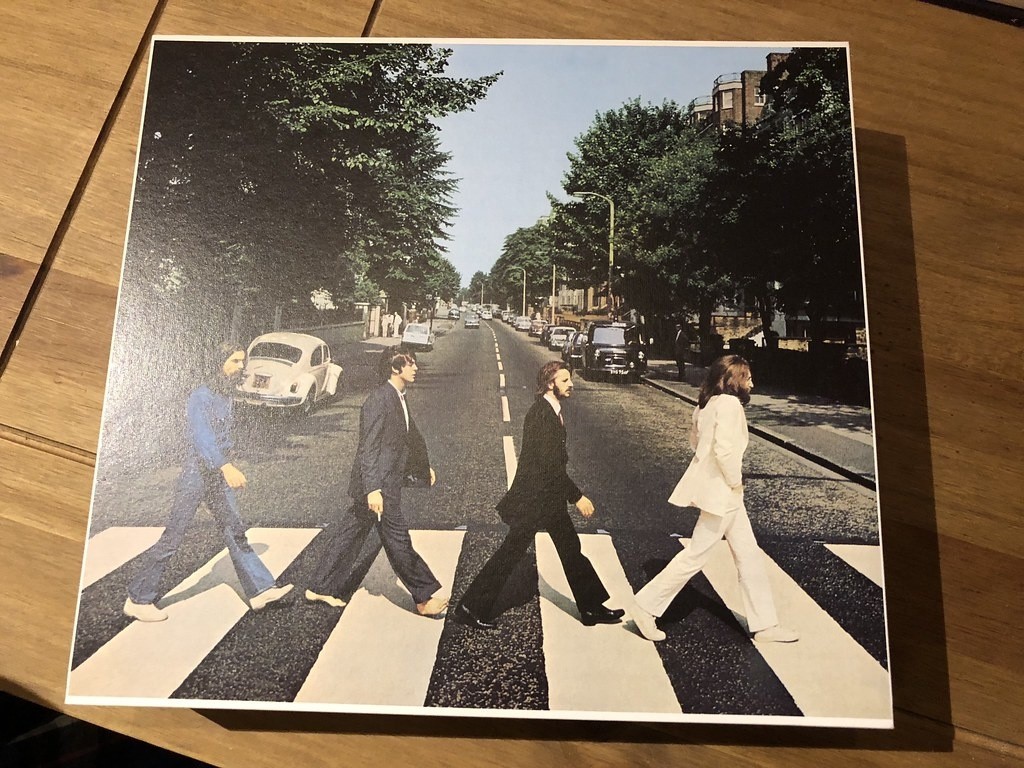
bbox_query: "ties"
[558,411,564,426]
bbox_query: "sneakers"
[627,601,666,641]
[754,626,800,643]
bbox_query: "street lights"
[512,266,526,317]
[572,191,616,316]
[535,251,556,326]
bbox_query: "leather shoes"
[581,606,625,626]
[249,583,294,610]
[123,598,168,623]
[454,602,496,630]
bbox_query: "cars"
[587,320,652,382]
[235,332,341,417]
[447,302,589,369]
[400,323,437,349]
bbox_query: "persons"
[673,324,691,381]
[454,361,625,629]
[123,342,294,623]
[381,311,402,338]
[626,355,800,644]
[305,344,450,616]
